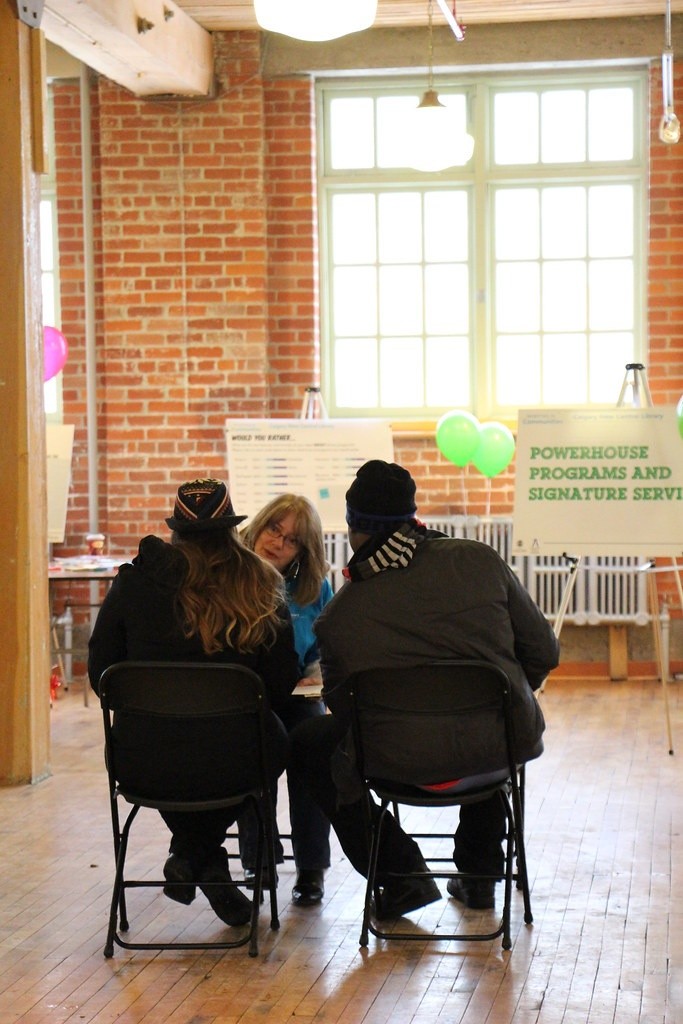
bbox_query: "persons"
[235,492,334,904]
[291,459,558,922]
[89,478,299,927]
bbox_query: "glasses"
[265,524,300,549]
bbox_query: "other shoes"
[196,869,253,927]
[164,853,197,906]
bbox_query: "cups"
[87,535,104,555]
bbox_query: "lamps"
[438,0,465,42]
[415,0,446,108]
[253,0,378,42]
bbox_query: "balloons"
[473,422,515,478]
[43,326,68,381]
[436,411,479,468]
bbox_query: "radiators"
[319,514,671,628]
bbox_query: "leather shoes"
[292,871,324,906]
[448,874,495,910]
[244,865,278,890]
[370,873,440,919]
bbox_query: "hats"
[347,460,417,536]
[165,477,247,532]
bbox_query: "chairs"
[92,659,282,959]
[345,656,533,951]
